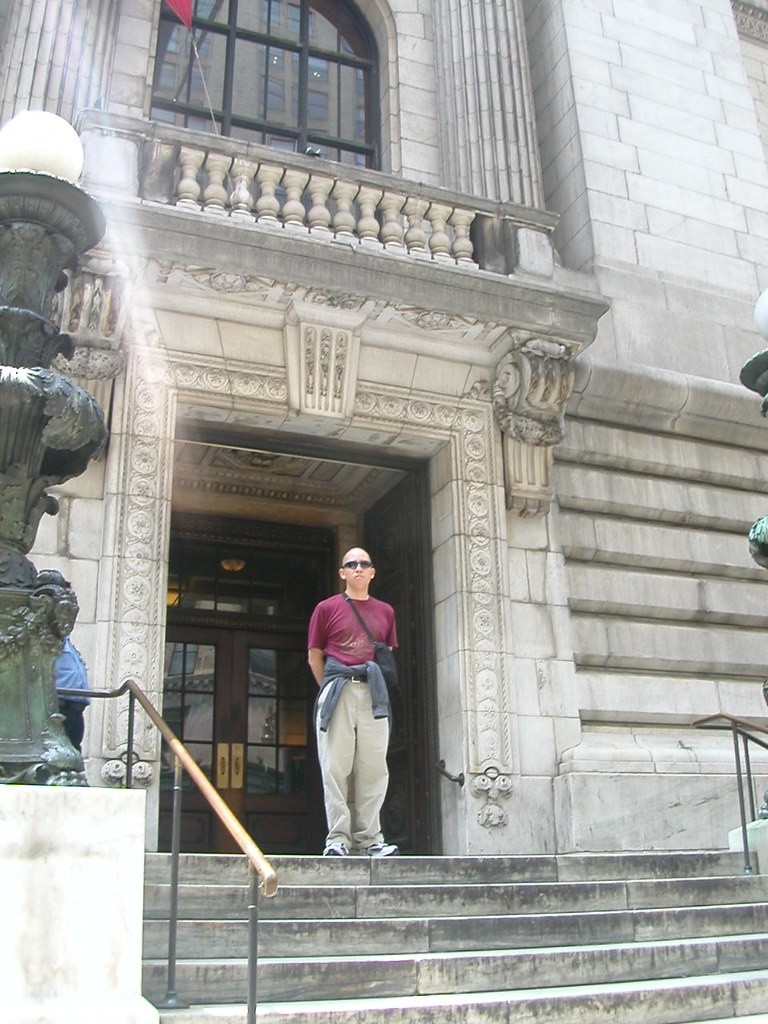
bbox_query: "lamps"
[219,556,246,572]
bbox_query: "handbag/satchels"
[375,644,399,689]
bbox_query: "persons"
[307,548,399,856]
[54,637,91,753]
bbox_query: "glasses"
[341,562,373,569]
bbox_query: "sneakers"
[323,842,348,856]
[356,842,398,856]
